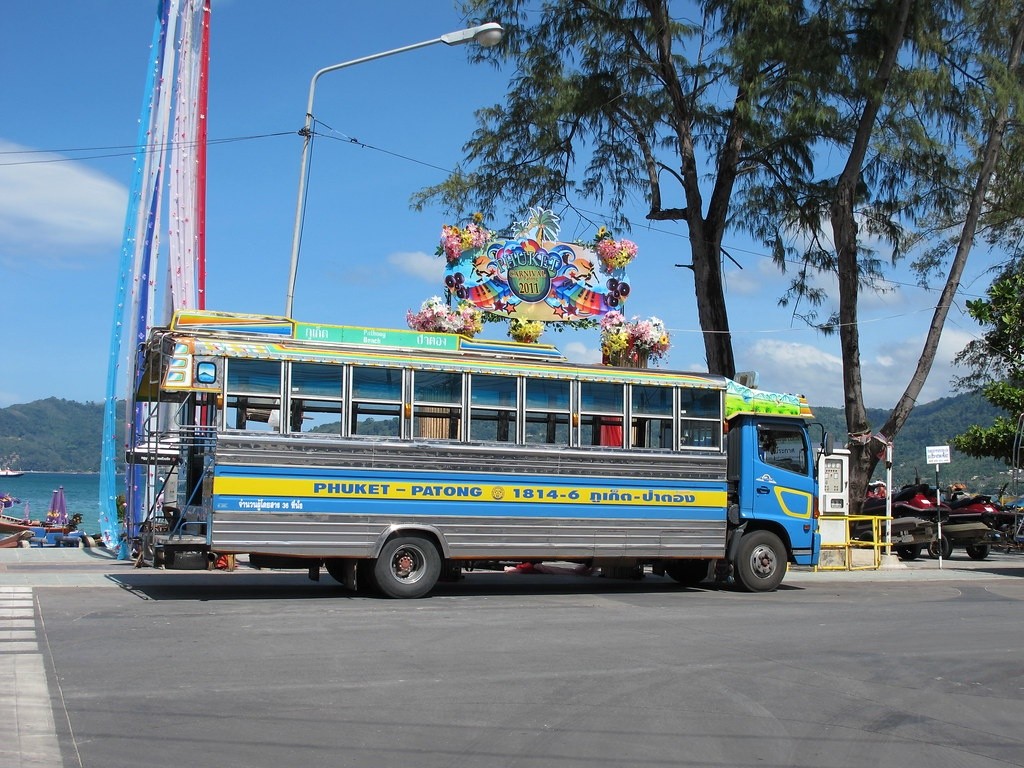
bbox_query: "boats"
[0,470,24,477]
[0,513,83,534]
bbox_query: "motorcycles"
[900,477,1015,560]
[854,480,952,561]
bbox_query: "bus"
[126,308,834,598]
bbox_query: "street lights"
[286,22,504,319]
